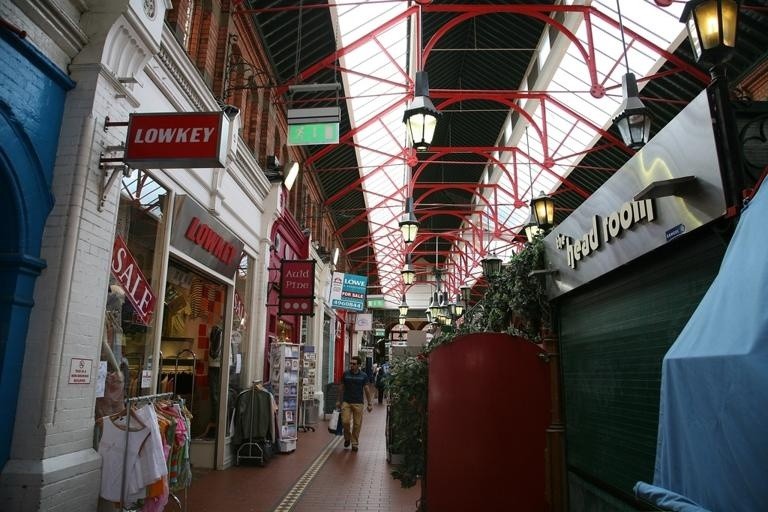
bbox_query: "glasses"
[349,362,358,365]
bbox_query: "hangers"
[95,392,193,433]
[240,382,274,399]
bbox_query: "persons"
[95,285,125,452]
[372,360,392,404]
[336,356,373,451]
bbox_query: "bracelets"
[368,404,372,406]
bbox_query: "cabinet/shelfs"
[269,342,300,454]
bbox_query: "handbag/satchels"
[328,411,343,436]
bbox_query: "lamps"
[402,5,444,153]
[401,253,416,286]
[330,247,341,266]
[398,293,409,326]
[398,166,421,247]
[610,0,654,150]
[681,0,741,79]
[524,190,555,245]
[480,251,503,286]
[283,161,300,190]
[424,286,472,327]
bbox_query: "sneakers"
[344,439,350,447]
[352,444,359,451]
